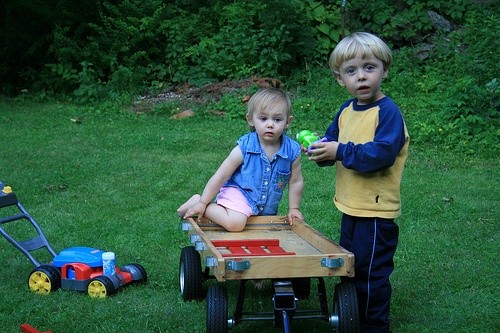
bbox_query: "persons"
[298,32,409,333]
[176,87,303,233]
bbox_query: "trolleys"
[179,212,360,333]
[0,181,147,298]
